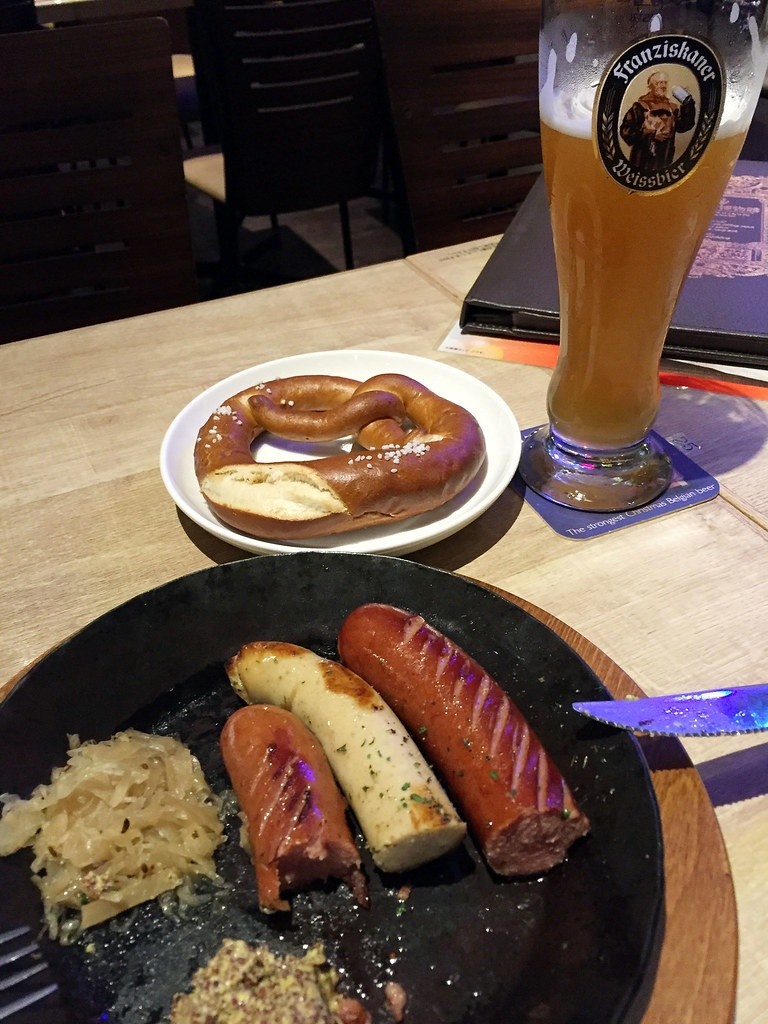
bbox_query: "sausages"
[336,602,590,878]
[224,639,466,872]
[220,704,362,916]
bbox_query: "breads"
[192,372,486,538]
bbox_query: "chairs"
[376,0,543,254]
[0,15,191,339]
[183,0,374,293]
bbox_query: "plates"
[159,349,522,555]
[0,553,664,1024]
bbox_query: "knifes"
[572,684,768,736]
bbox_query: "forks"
[0,926,58,1019]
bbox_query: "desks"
[0,235,768,1024]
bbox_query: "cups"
[525,0,768,512]
